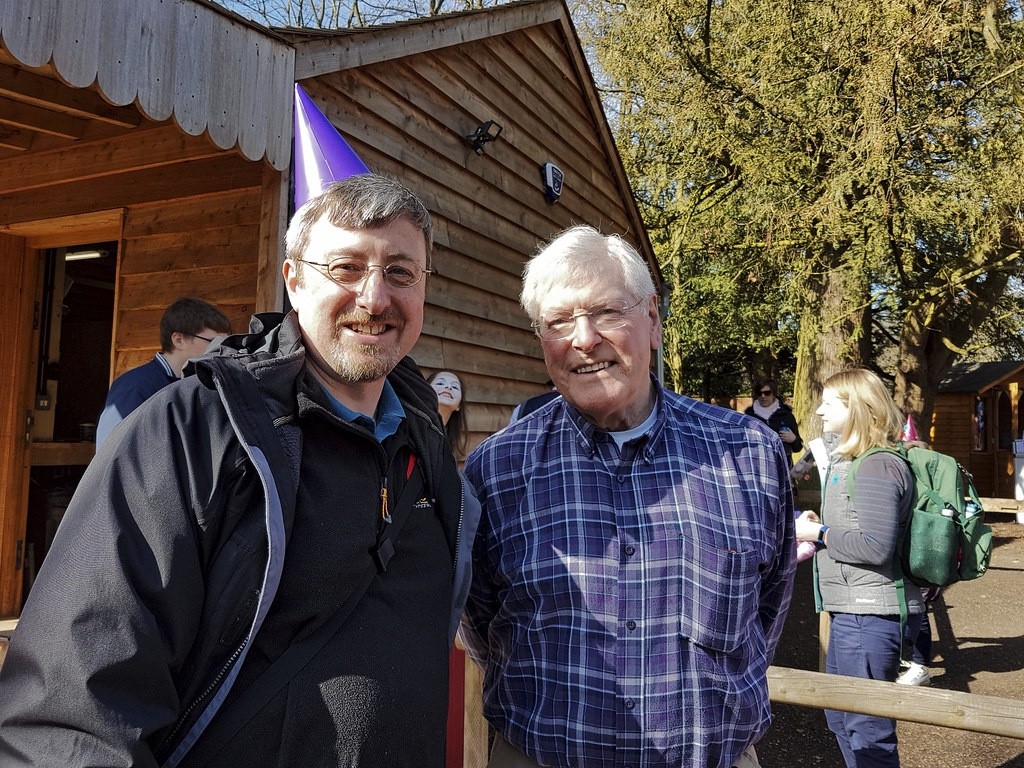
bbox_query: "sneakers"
[896,660,931,686]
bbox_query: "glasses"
[756,391,773,397]
[297,256,431,289]
[529,296,645,341]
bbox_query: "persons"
[897,414,931,685]
[796,370,925,768]
[458,224,798,768]
[510,379,561,425]
[97,298,232,453]
[744,378,804,496]
[427,370,470,456]
[0,81,485,768]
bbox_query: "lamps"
[466,119,503,156]
[65,250,110,261]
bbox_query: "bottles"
[779,421,787,431]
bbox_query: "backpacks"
[849,441,993,588]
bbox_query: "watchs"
[818,525,830,545]
